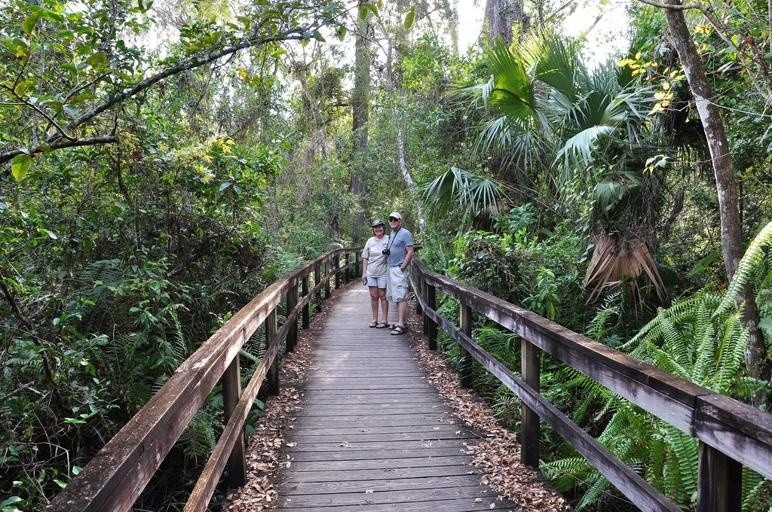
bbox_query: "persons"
[362,219,390,328]
[386,212,415,335]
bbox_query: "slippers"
[369,320,407,335]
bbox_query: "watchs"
[400,267,406,272]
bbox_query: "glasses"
[390,219,398,222]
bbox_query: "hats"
[389,212,402,220]
[371,218,385,227]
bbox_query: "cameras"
[382,248,390,256]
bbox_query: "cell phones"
[364,281,366,285]
